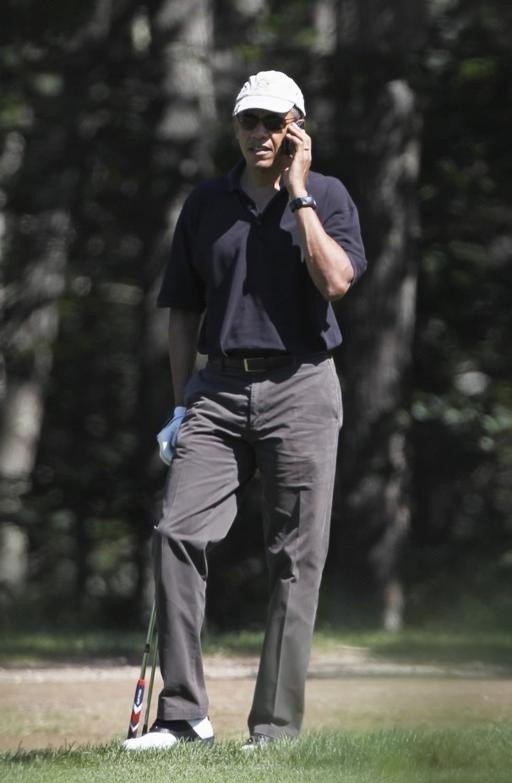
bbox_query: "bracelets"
[288,196,318,213]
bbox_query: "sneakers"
[125,714,214,753]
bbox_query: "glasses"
[235,114,295,131]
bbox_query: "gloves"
[155,406,186,466]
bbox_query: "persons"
[120,69,369,754]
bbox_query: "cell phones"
[285,119,305,156]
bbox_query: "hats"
[231,70,307,119]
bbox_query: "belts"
[208,350,319,374]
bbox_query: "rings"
[304,147,309,151]
